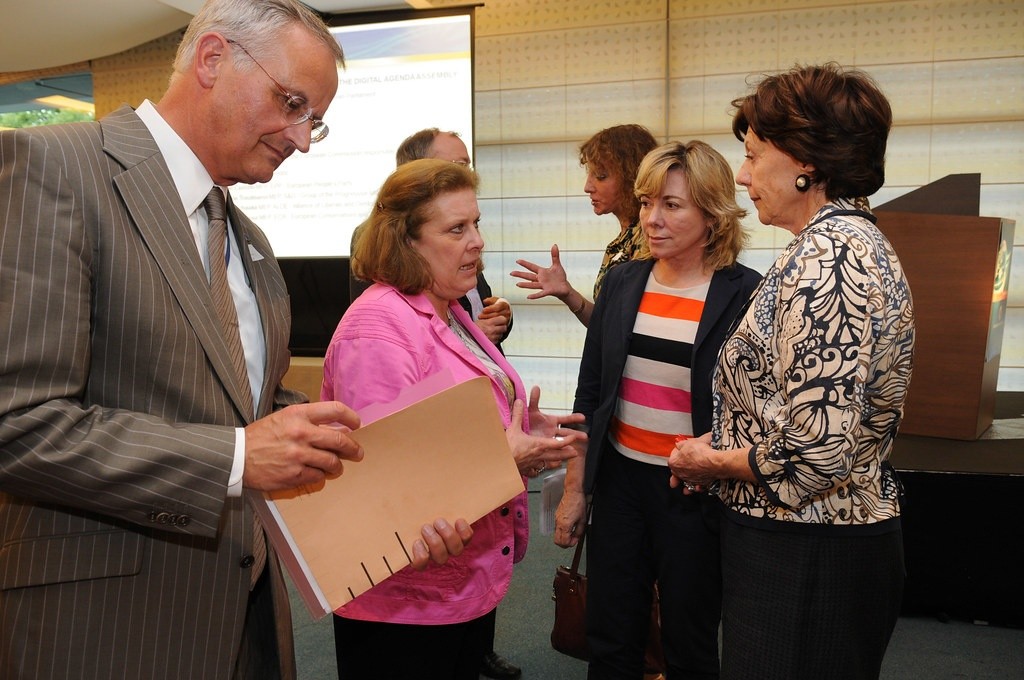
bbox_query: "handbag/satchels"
[551,564,666,675]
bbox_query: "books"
[244,375,525,621]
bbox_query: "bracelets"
[573,296,585,316]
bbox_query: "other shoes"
[483,652,521,680]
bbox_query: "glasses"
[226,40,329,143]
[449,158,474,171]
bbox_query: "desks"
[887,391,1024,632]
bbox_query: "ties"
[204,186,266,591]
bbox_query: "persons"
[509,123,663,680]
[552,140,766,679]
[0,0,475,680]
[318,127,588,680]
[669,63,915,680]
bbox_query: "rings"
[537,460,547,473]
